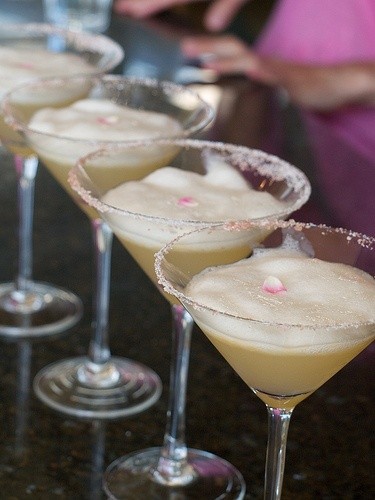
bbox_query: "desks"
[0,0,375,500]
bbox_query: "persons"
[113,0,375,165]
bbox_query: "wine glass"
[0,22,124,340]
[0,74,215,417]
[153,218,375,500]
[67,139,312,500]
